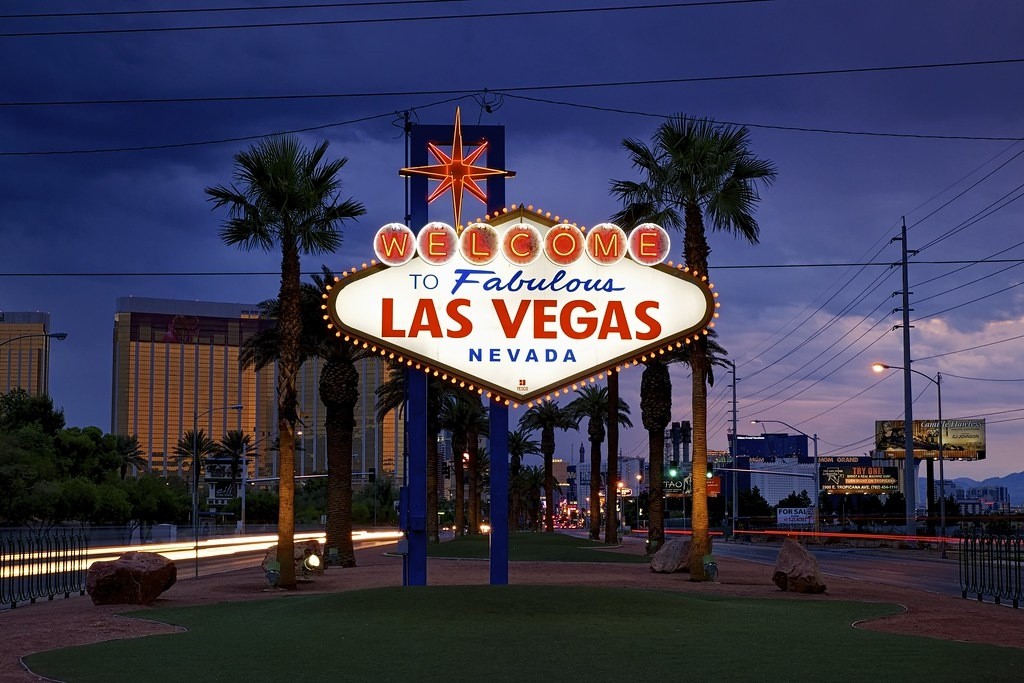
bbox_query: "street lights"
[750,419,820,545]
[872,363,947,560]
[193,403,245,539]
[242,425,303,534]
[619,482,640,537]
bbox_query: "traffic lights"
[445,466,451,479]
[670,461,677,478]
[441,462,447,475]
[707,462,713,479]
